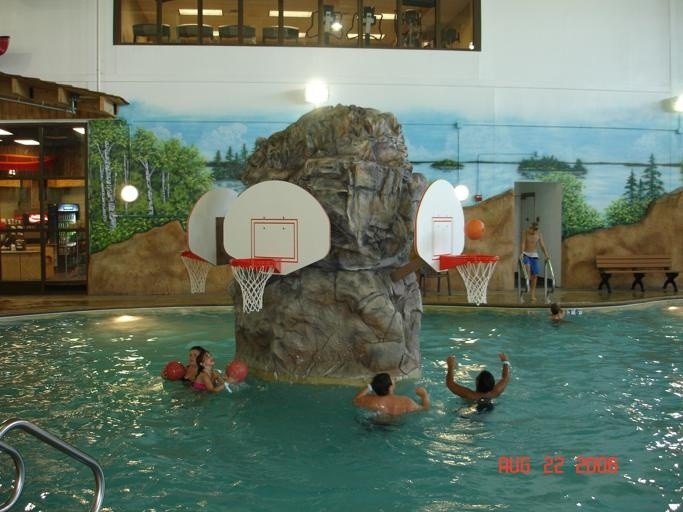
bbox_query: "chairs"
[419,270,450,297]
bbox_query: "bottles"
[58,213,76,247]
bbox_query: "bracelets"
[366,383,373,392]
[224,381,228,387]
[502,360,510,365]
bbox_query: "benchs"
[595,254,679,292]
[132,24,300,44]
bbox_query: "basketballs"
[226,361,247,380]
[166,361,186,380]
[464,219,484,239]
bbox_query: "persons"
[547,302,565,320]
[191,350,240,397]
[518,223,550,301]
[160,346,206,383]
[352,373,430,431]
[446,352,510,411]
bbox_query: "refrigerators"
[48,203,80,273]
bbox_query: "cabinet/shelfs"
[0,246,58,281]
